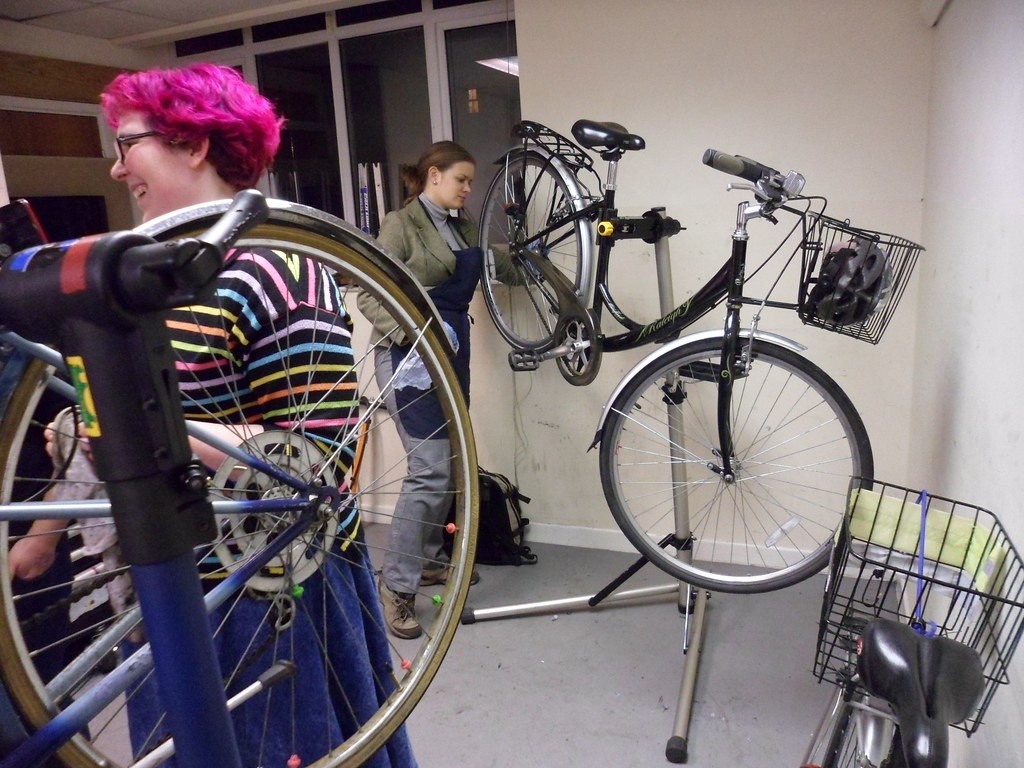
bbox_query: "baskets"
[799,208,925,345]
[812,475,1024,739]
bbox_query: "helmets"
[806,236,889,325]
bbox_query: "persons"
[0,65,417,768]
[356,140,546,639]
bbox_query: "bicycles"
[0,186,476,768]
[478,119,925,594]
[798,478,1024,768]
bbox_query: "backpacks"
[445,463,538,568]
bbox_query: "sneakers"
[419,564,480,586]
[378,580,422,639]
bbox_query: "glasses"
[114,131,162,163]
